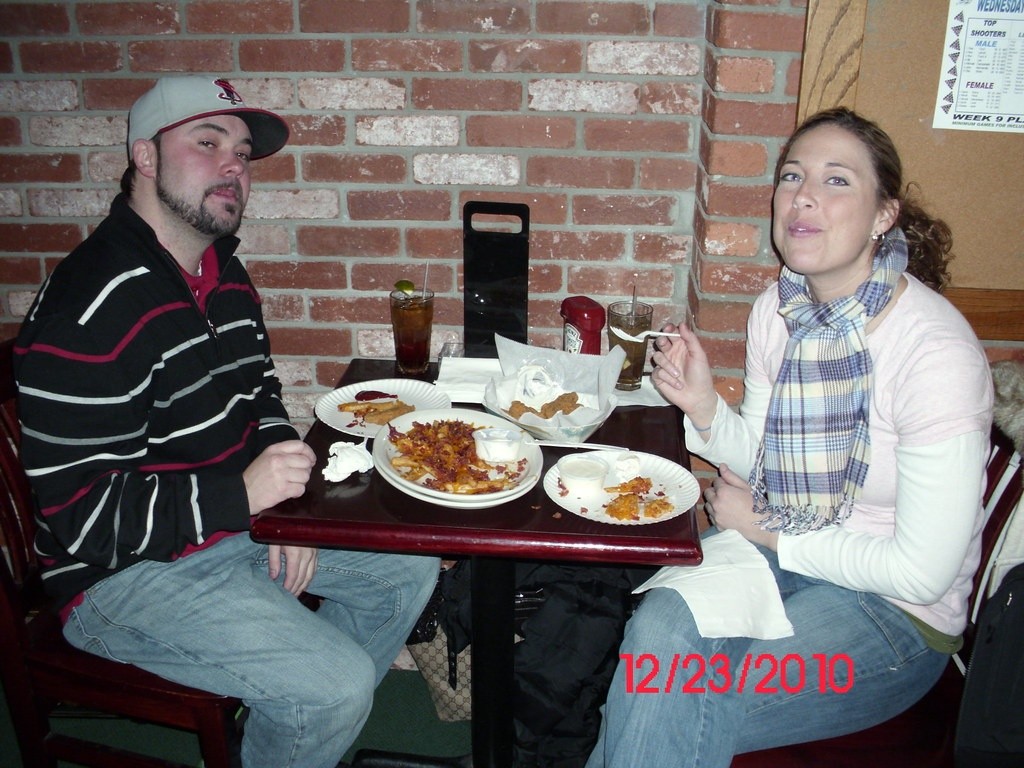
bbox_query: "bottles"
[560,296,606,355]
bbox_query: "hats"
[128,76,290,161]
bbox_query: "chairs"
[1,336,321,768]
[730,360,1024,768]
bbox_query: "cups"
[390,287,435,374]
[607,301,654,392]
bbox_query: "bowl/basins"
[472,428,523,461]
[557,455,609,502]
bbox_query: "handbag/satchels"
[406,557,636,766]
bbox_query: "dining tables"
[251,359,703,768]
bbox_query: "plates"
[314,378,452,438]
[372,408,544,510]
[543,450,702,527]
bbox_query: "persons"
[0,74,441,768]
[585,106,995,768]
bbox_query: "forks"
[610,325,682,342]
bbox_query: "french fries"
[389,431,439,482]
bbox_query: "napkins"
[435,357,504,402]
[631,527,796,640]
[613,376,672,407]
[322,435,374,482]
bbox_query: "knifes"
[525,439,630,452]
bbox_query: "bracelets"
[692,423,711,432]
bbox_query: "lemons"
[394,279,414,296]
